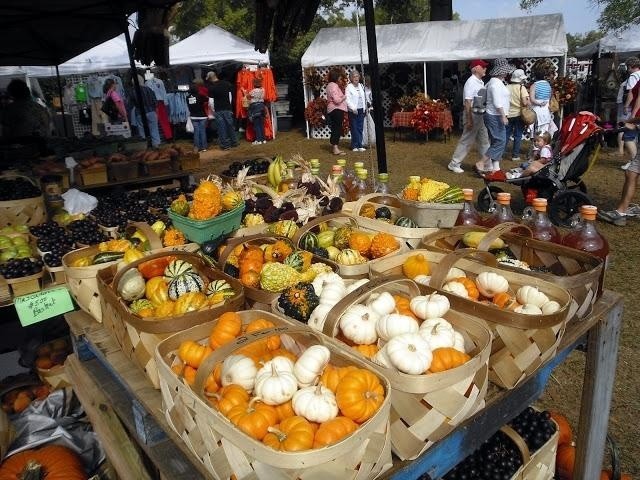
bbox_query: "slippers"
[331,152,346,155]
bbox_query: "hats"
[489,58,517,76]
[510,69,528,82]
[469,60,489,69]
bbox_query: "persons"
[0,79,50,137]
[326,70,347,155]
[104,70,173,152]
[448,56,640,227]
[186,86,209,152]
[345,71,367,152]
[205,71,239,150]
[241,80,267,145]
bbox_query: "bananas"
[267,152,287,187]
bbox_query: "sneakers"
[252,140,267,145]
[598,205,640,226]
[353,148,367,152]
[448,156,521,178]
[621,163,631,170]
[608,151,624,157]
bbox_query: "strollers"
[478,111,627,230]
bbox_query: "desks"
[80,168,208,187]
[392,111,453,143]
[64,290,623,480]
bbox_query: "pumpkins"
[557,442,577,480]
[36,340,67,369]
[69,179,573,453]
[600,468,633,480]
[0,444,85,480]
[2,384,50,413]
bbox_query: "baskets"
[63,192,603,480]
[222,157,274,186]
[0,174,47,227]
[65,195,603,480]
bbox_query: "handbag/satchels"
[472,89,486,108]
[520,107,536,124]
[206,71,214,80]
[101,96,118,119]
[622,86,629,104]
[548,95,560,112]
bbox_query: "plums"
[1,176,199,279]
[223,156,270,176]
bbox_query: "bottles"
[454,188,609,305]
[278,158,399,210]
